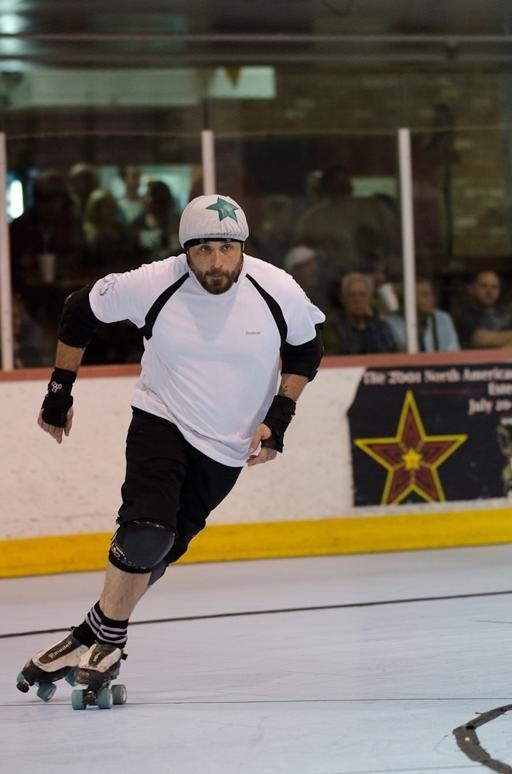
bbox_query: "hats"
[285,247,314,269]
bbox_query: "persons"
[0,133,512,356]
[15,194,336,709]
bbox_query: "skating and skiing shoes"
[71,643,127,708]
[17,628,90,700]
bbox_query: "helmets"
[178,195,249,248]
